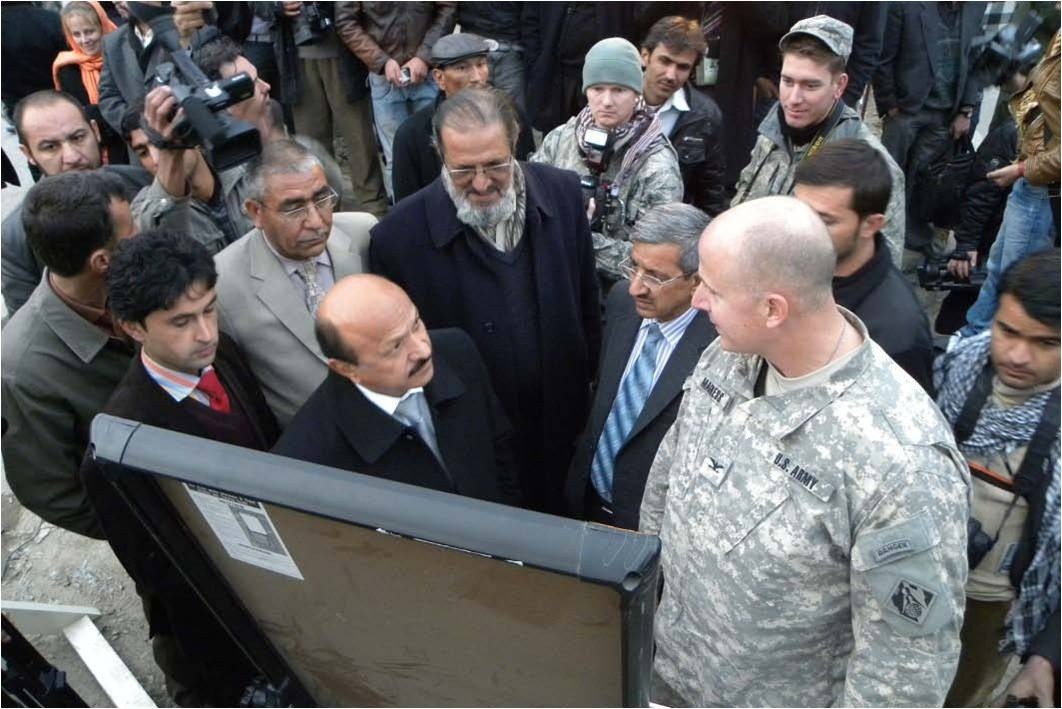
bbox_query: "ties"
[294,261,327,319]
[395,392,453,485]
[589,322,664,502]
[196,370,231,414]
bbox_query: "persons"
[1,2,1061,708]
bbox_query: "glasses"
[438,150,512,185]
[617,256,698,291]
[260,187,340,225]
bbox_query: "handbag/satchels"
[908,134,978,229]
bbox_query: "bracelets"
[1016,160,1026,177]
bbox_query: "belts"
[587,489,637,522]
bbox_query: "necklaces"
[823,317,847,369]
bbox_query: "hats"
[430,33,492,65]
[779,14,854,65]
[583,36,644,96]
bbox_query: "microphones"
[178,95,227,146]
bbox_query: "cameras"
[916,251,988,291]
[581,127,616,232]
[400,69,410,83]
